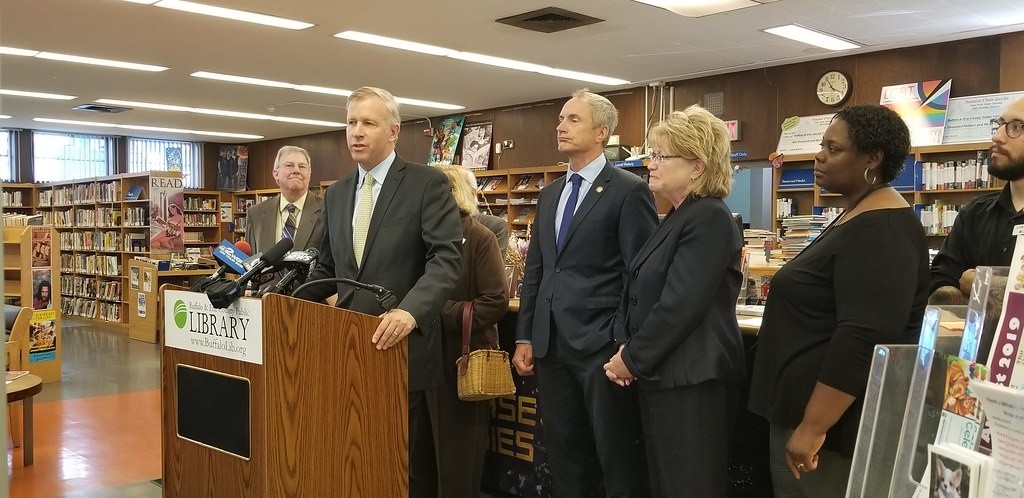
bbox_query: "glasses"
[989,119,1024,139]
[651,151,683,162]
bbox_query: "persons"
[218,149,226,189]
[296,86,462,498]
[231,148,241,188]
[245,146,337,308]
[512,88,659,498]
[466,169,509,261]
[747,105,929,498]
[930,97,1024,306]
[425,164,509,498]
[151,204,184,250]
[603,104,744,498]
[33,280,51,310]
[223,149,232,187]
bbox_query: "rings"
[394,332,398,336]
[798,463,804,468]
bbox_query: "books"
[0,145,1024,498]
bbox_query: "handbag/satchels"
[455,299,517,402]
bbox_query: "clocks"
[814,69,853,107]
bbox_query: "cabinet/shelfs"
[0,182,35,226]
[477,168,509,223]
[36,171,183,331]
[183,189,223,261]
[815,148,917,231]
[3,225,61,383]
[915,141,1007,237]
[510,161,570,254]
[126,258,219,345]
[230,188,281,242]
[769,151,817,247]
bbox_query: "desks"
[748,264,783,276]
[5,370,44,467]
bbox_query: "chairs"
[5,302,35,372]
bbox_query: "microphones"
[189,237,321,310]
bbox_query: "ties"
[557,174,584,252]
[281,204,296,258]
[353,173,376,269]
